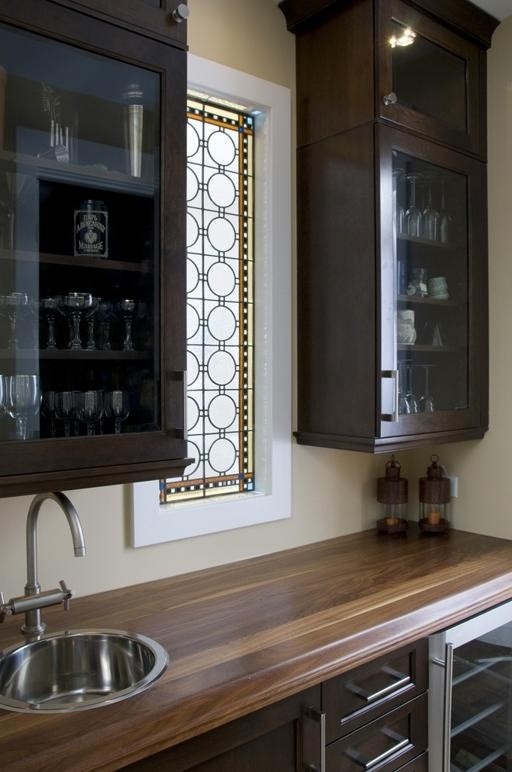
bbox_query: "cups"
[397,308,418,346]
[427,276,448,301]
[407,268,428,298]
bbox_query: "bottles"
[69,190,112,259]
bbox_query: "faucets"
[0,490,86,637]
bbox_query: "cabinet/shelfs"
[110,683,321,771]
[430,600,511,771]
[321,636,430,772]
[75,1,190,42]
[0,0,189,498]
[278,1,501,164]
[291,120,491,456]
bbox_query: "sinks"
[0,628,170,715]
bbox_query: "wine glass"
[393,168,458,247]
[3,289,148,351]
[1,373,131,435]
[398,358,437,415]
